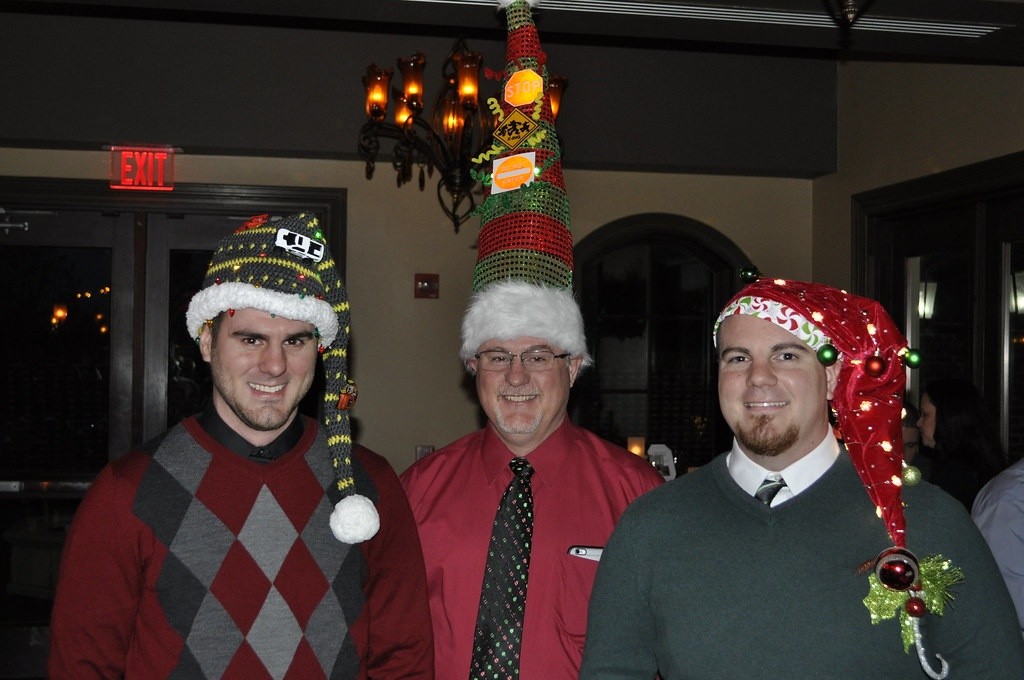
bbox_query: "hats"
[712,262,966,547]
[185,211,380,543]
[459,2,592,372]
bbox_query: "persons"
[577,280,1024,680]
[48,210,434,680]
[398,267,666,680]
[896,374,983,515]
[971,457,1024,637]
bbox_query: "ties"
[755,477,789,506]
[469,457,535,680]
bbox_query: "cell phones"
[567,545,604,562]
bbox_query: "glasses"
[475,351,571,371]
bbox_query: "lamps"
[355,41,580,234]
